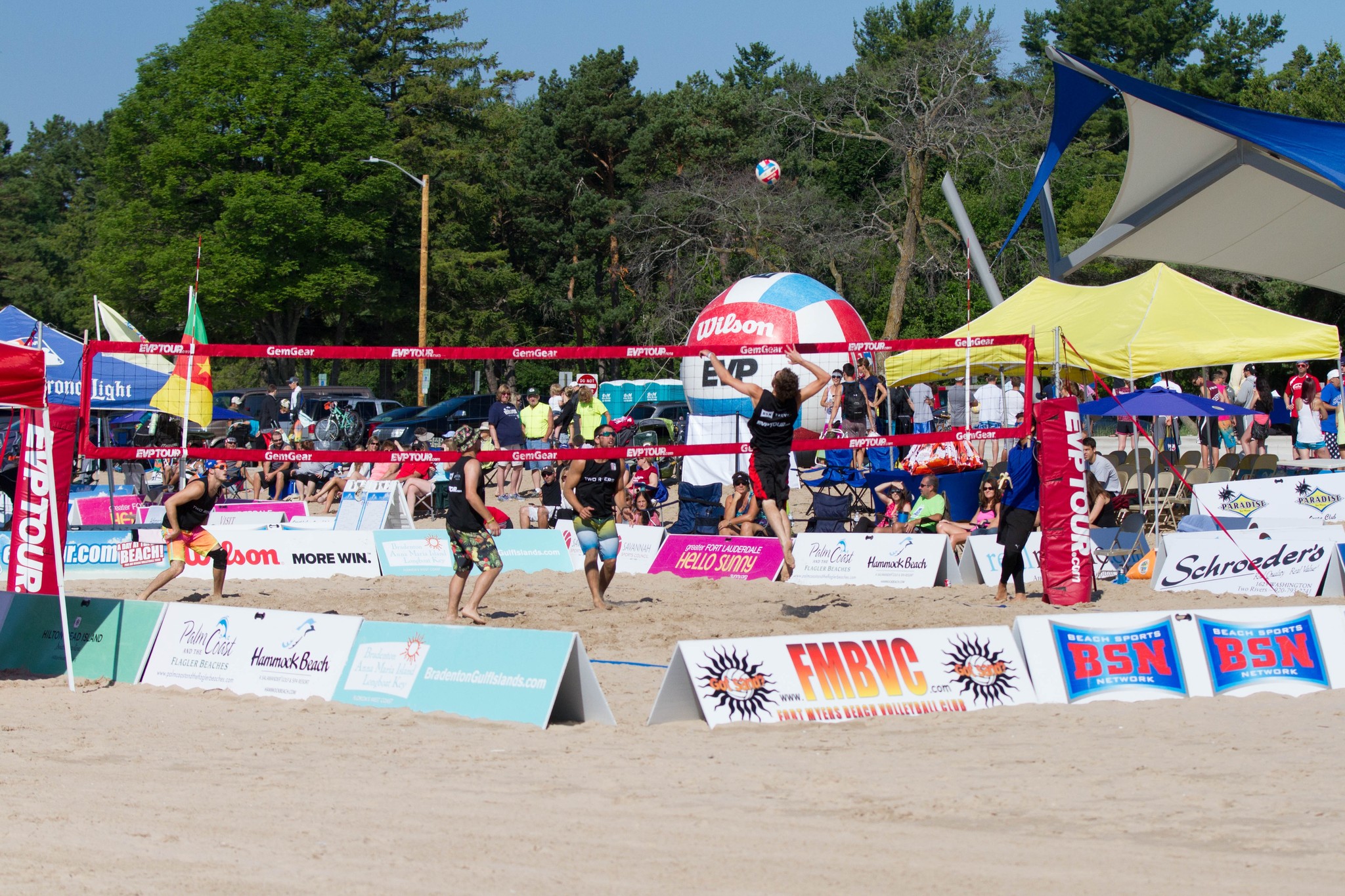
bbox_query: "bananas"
[998,472,1013,489]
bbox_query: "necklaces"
[210,482,221,498]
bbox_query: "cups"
[338,466,342,471]
[855,377,860,382]
[640,481,646,491]
[897,511,909,523]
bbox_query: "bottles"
[198,464,204,474]
[889,510,898,527]
[139,411,152,425]
[787,510,793,520]
[1117,564,1126,585]
[829,397,834,412]
[334,471,339,477]
[853,512,860,528]
[148,412,159,435]
[704,507,712,518]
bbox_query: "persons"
[136,459,228,603]
[717,471,776,539]
[700,344,830,580]
[446,425,503,624]
[112,365,662,529]
[991,411,1038,605]
[821,342,1345,549]
[563,424,636,610]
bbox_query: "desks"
[865,465,986,522]
[1276,458,1345,473]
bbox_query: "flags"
[149,291,213,427]
[97,300,175,373]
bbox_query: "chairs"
[917,489,953,534]
[804,492,853,533]
[836,446,899,516]
[1094,448,1279,537]
[790,446,863,512]
[414,480,450,522]
[632,462,669,527]
[121,462,175,506]
[311,462,344,500]
[1093,513,1146,582]
[666,482,725,535]
[221,461,248,501]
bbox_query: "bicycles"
[314,396,367,449]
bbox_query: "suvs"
[622,399,690,441]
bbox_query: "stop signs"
[577,374,598,396]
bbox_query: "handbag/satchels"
[1111,492,1146,510]
[1250,420,1269,440]
[1163,425,1180,456]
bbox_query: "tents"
[0,304,255,420]
[883,261,1345,527]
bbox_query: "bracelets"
[486,517,494,524]
[620,506,628,515]
[706,352,715,359]
[728,519,732,527]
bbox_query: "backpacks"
[133,412,179,449]
[841,382,867,421]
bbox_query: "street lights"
[363,156,431,407]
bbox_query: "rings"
[719,525,720,527]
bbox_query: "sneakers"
[529,491,539,498]
[497,493,526,502]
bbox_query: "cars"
[363,393,553,447]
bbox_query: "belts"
[526,437,544,441]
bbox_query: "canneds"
[640,482,646,491]
[944,579,951,587]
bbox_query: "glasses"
[542,472,555,476]
[502,393,511,396]
[228,441,237,445]
[371,442,380,446]
[734,480,747,486]
[439,447,444,451]
[1192,379,1197,385]
[1296,364,1307,368]
[273,438,282,442]
[385,450,394,452]
[562,475,567,479]
[1015,422,1022,427]
[890,489,902,494]
[412,448,423,451]
[637,458,644,460]
[832,375,841,378]
[596,431,616,438]
[920,484,932,488]
[288,381,293,384]
[983,487,993,491]
[857,365,861,368]
[211,464,229,470]
[774,371,779,381]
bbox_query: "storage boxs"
[269,478,299,501]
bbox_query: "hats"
[541,465,555,475]
[955,377,964,381]
[1340,356,1345,365]
[1035,384,1054,399]
[527,387,538,396]
[1244,363,1257,373]
[201,458,226,476]
[569,381,579,387]
[231,396,242,405]
[1296,360,1309,365]
[286,377,297,382]
[1327,369,1345,381]
[412,421,490,453]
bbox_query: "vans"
[1226,355,1345,400]
[906,387,951,424]
[283,395,407,454]
[177,386,378,462]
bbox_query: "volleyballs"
[755,160,781,185]
[682,271,878,440]
